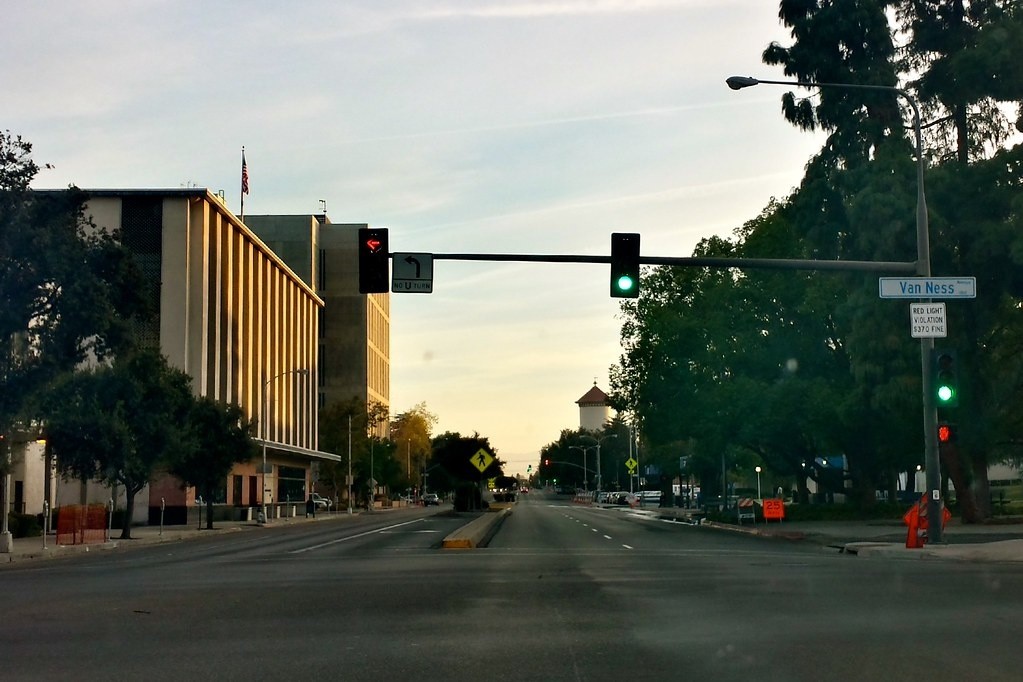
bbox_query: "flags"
[241,150,249,195]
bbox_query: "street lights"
[568,445,602,494]
[580,434,617,504]
[347,412,376,514]
[262,369,309,521]
[726,72,943,546]
[755,466,762,501]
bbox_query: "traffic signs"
[878,275,976,302]
[391,252,433,294]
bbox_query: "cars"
[598,483,701,503]
[488,484,529,493]
[309,492,332,507]
[424,494,439,506]
[309,498,327,511]
[402,495,421,504]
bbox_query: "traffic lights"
[610,233,640,300]
[358,228,389,294]
[928,346,959,410]
[937,421,957,447]
[545,459,549,466]
